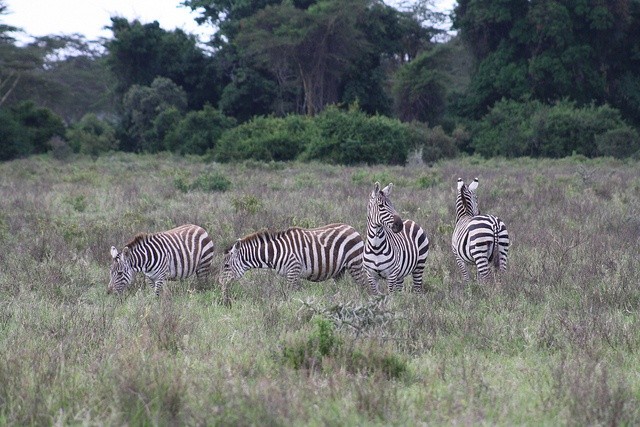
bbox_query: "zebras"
[452,177,510,286]
[362,181,429,297]
[107,224,214,298]
[218,223,366,290]
